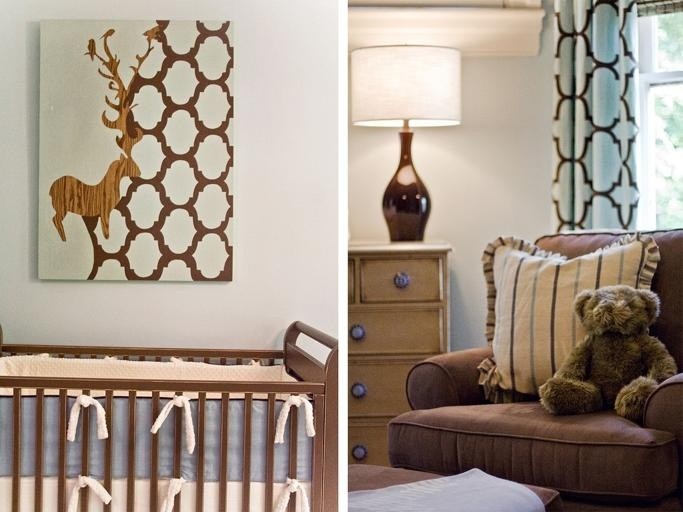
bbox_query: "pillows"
[481,232,660,403]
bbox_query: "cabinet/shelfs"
[348,243,452,466]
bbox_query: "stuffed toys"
[537,283,678,425]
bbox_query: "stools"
[349,464,562,512]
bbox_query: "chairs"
[387,228,683,512]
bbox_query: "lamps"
[350,45,463,243]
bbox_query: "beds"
[0,320,338,512]
